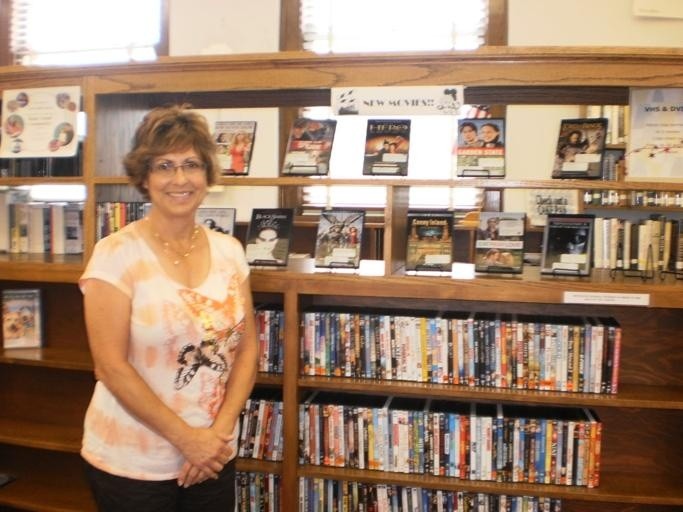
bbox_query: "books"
[639,219,653,271]
[236,397,284,461]
[64,203,80,254]
[664,220,670,270]
[654,221,660,271]
[551,118,608,179]
[79,210,83,255]
[7,159,79,177]
[585,190,683,207]
[281,118,337,175]
[650,213,666,271]
[245,207,293,270]
[618,220,623,267]
[2,289,42,348]
[541,213,592,275]
[94,203,142,241]
[476,211,526,274]
[0,189,30,254]
[639,224,648,271]
[315,211,364,268]
[631,224,638,270]
[235,469,280,512]
[626,87,683,183]
[28,208,50,254]
[457,118,506,179]
[610,217,618,269]
[20,199,45,255]
[195,208,236,236]
[670,220,679,270]
[603,219,609,269]
[679,219,683,270]
[51,205,66,255]
[363,120,410,176]
[212,121,256,175]
[9,202,20,253]
[298,476,562,512]
[594,217,602,269]
[623,219,631,271]
[406,210,453,276]
[254,301,284,373]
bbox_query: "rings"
[222,445,228,453]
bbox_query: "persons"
[213,133,230,171]
[348,228,358,247]
[375,140,389,162]
[204,219,215,229]
[4,114,25,153]
[55,93,75,110]
[214,226,223,231]
[558,130,589,162]
[48,123,74,151]
[249,218,284,266]
[79,103,258,512]
[390,144,396,153]
[459,122,484,165]
[480,122,504,148]
[228,130,249,173]
[482,217,514,268]
[7,93,28,113]
[560,228,586,254]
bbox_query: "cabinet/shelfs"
[0,274,284,512]
[0,79,86,265]
[292,294,663,512]
[89,54,683,306]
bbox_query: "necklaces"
[142,215,199,266]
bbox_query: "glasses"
[149,160,207,175]
